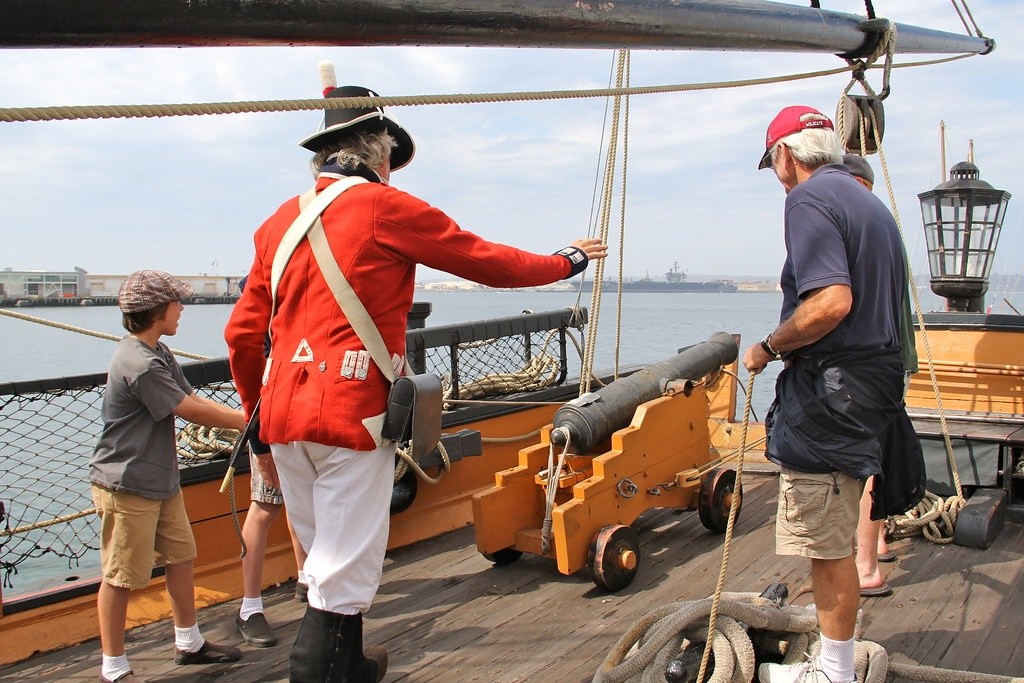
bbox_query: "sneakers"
[235,607,278,648]
[759,656,859,683]
[98,671,147,683]
[295,582,310,602]
[362,644,388,683]
[174,640,243,665]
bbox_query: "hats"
[299,85,416,175]
[757,104,835,170]
[119,269,195,314]
[842,154,874,185]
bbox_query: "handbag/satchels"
[878,406,926,516]
[381,372,443,463]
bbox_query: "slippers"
[860,550,896,597]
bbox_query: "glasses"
[763,147,775,169]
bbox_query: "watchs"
[761,333,779,356]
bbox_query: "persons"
[87,268,250,683]
[223,57,609,682]
[236,273,308,649]
[835,155,920,598]
[743,105,905,682]
